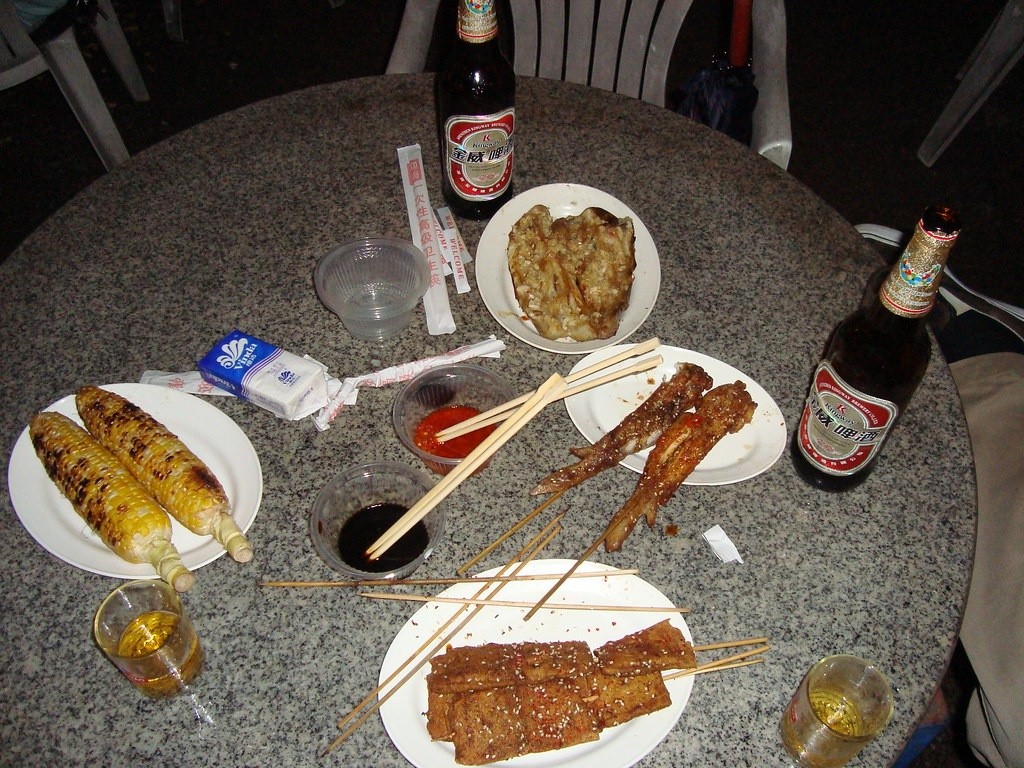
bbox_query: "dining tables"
[0,67,976,768]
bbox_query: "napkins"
[197,331,329,417]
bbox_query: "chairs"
[383,2,792,175]
[0,0,151,175]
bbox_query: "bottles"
[435,0,516,219]
[790,198,963,489]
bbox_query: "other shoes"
[854,223,1024,342]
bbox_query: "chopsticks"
[436,336,663,443]
[365,371,568,561]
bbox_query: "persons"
[921,355,1024,768]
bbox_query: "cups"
[93,579,206,696]
[780,654,894,768]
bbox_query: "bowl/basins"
[314,235,432,339]
[392,362,516,477]
[309,460,446,581]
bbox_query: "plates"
[8,384,263,579]
[378,557,697,768]
[563,344,787,486]
[474,182,661,352]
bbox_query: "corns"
[30,385,254,592]
